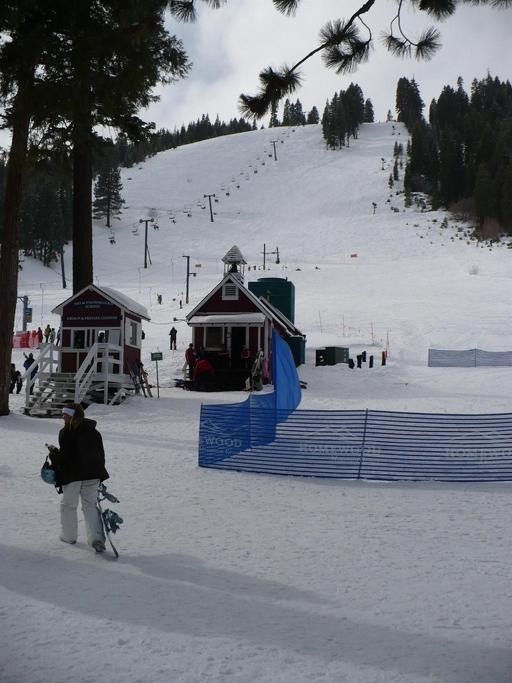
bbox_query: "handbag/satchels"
[41,462,56,484]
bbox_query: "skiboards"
[125,357,154,398]
[299,380,307,390]
[23,352,28,359]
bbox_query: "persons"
[49,328,56,348]
[98,332,108,343]
[234,344,264,391]
[185,343,220,392]
[56,327,60,349]
[169,326,177,351]
[23,354,38,396]
[45,404,108,555]
[36,327,43,343]
[27,330,36,347]
[44,324,51,343]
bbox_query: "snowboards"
[96,482,122,559]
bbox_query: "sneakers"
[60,536,76,544]
[92,540,106,552]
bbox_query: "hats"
[62,403,84,418]
